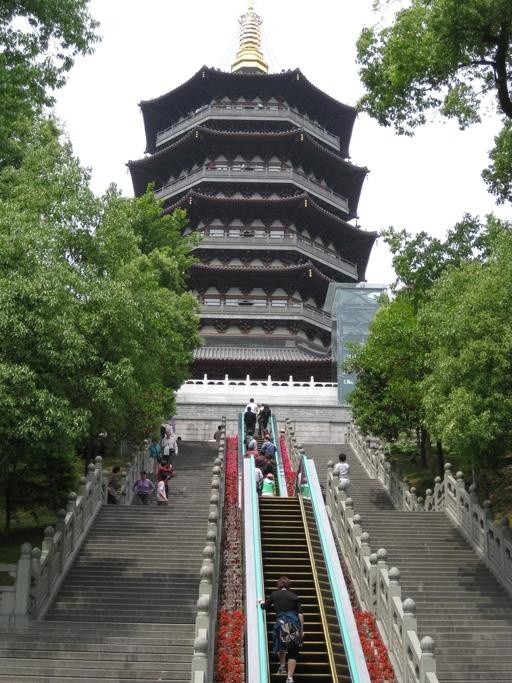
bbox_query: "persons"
[244,396,278,497]
[332,452,351,490]
[160,429,178,462]
[157,460,172,498]
[131,469,154,505]
[156,471,169,504]
[148,438,162,474]
[106,465,123,504]
[213,425,222,451]
[255,576,306,683]
